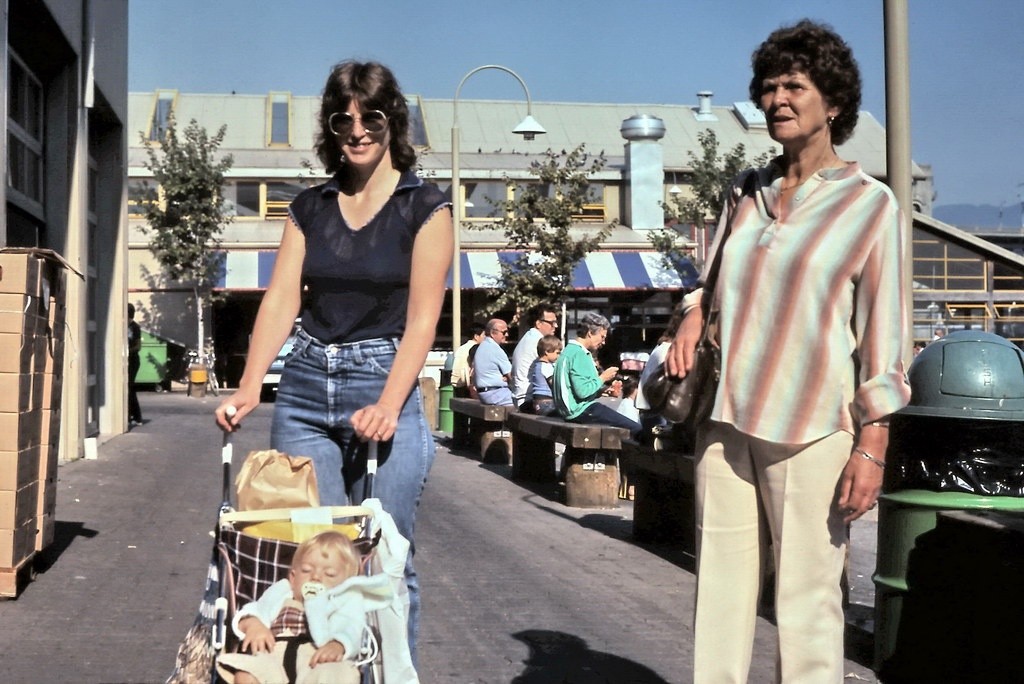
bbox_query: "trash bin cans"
[439,385,455,432]
[135,330,168,385]
[874,330,1023,684]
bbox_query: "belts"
[477,386,509,392]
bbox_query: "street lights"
[452,64,549,444]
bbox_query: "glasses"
[328,110,393,136]
[488,327,508,335]
[540,319,559,327]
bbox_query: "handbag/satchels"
[642,340,721,429]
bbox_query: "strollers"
[212,403,385,684]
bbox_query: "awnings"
[211,248,699,293]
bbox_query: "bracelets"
[854,446,886,470]
[866,419,890,429]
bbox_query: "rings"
[375,431,383,438]
[868,504,874,510]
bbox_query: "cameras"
[604,371,629,386]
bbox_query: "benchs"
[505,411,630,509]
[448,397,520,466]
[620,437,695,548]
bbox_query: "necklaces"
[780,156,840,196]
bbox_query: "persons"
[127,303,144,426]
[553,311,643,446]
[215,58,455,684]
[216,530,395,684]
[528,333,563,416]
[663,17,912,684]
[451,319,514,406]
[511,303,558,417]
[634,282,705,456]
[913,329,945,357]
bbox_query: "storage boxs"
[0,251,68,569]
